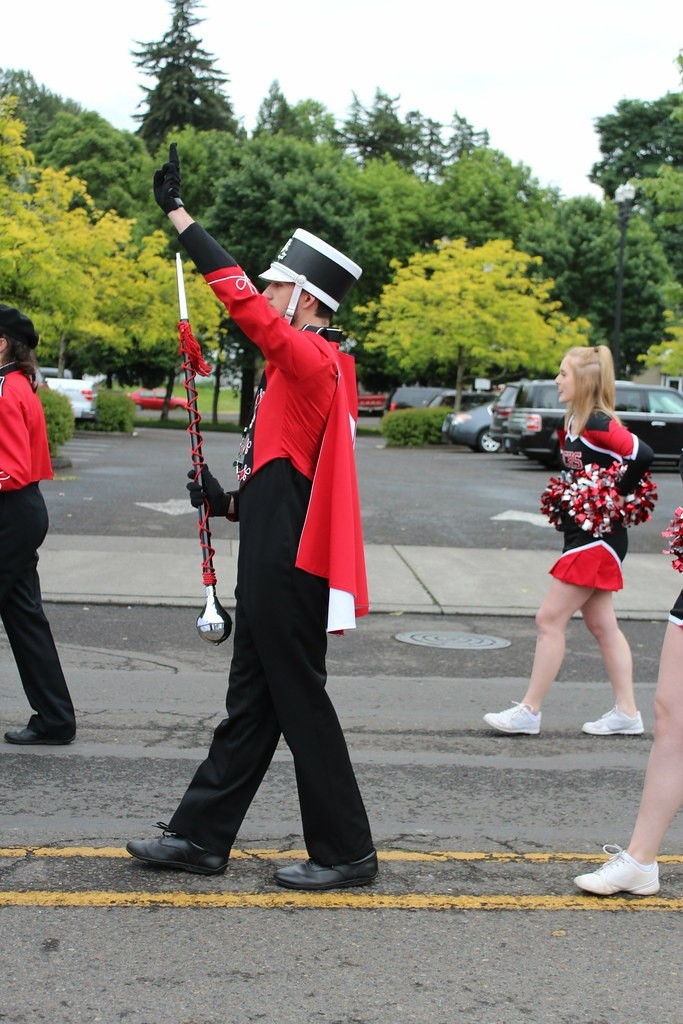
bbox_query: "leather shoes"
[274,848,379,889]
[125,821,229,875]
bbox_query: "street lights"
[611,182,636,377]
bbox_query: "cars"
[441,401,502,453]
[127,388,188,410]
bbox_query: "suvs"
[489,379,683,469]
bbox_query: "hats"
[257,228,364,312]
[0,303,40,349]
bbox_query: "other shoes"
[4,727,76,744]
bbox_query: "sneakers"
[582,704,645,735]
[572,843,660,895]
[483,700,542,734]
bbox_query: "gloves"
[152,142,185,217]
[186,463,232,517]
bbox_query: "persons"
[575,593,683,893]
[124,142,376,888]
[483,344,653,740]
[0,305,75,747]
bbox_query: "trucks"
[43,377,99,421]
